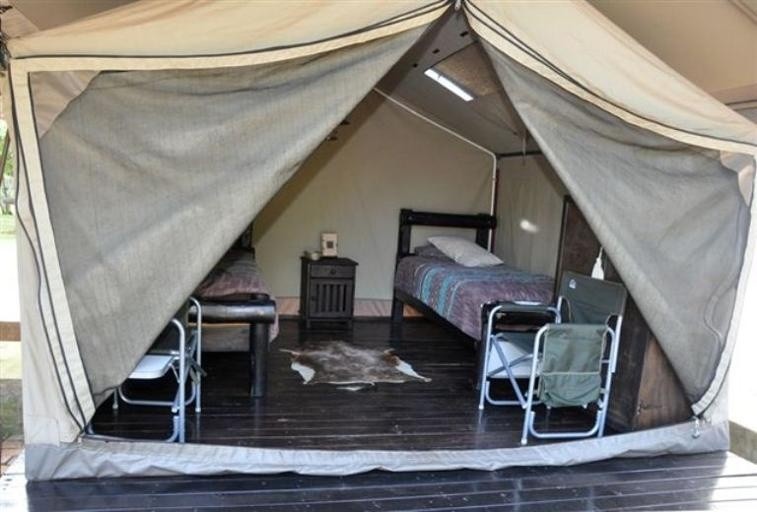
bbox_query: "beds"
[391,208,556,377]
[188,221,279,398]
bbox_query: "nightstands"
[298,256,359,332]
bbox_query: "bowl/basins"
[304,250,321,262]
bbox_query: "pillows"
[415,245,451,261]
[427,236,504,268]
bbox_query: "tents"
[4,1,757,482]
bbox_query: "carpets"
[279,339,432,393]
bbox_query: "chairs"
[79,296,202,444]
[479,268,627,445]
